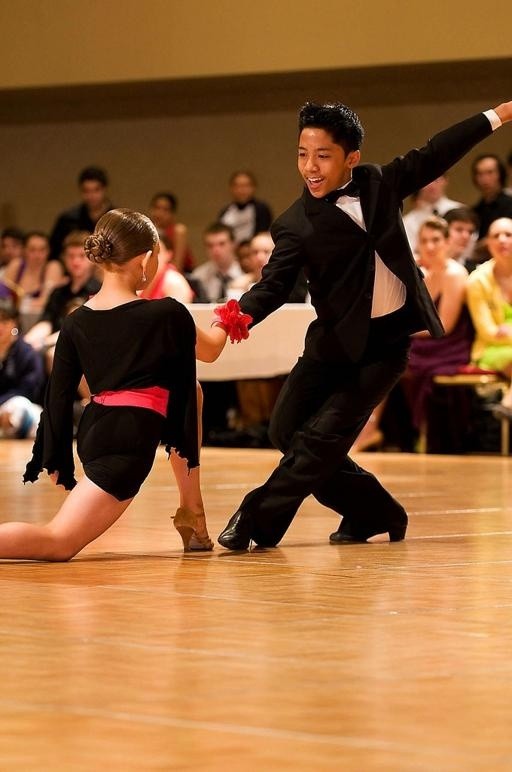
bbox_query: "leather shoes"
[217,507,251,551]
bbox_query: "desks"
[187,302,317,383]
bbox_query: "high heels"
[170,507,215,554]
[329,514,408,545]
[351,432,384,453]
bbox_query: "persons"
[217,100,512,554]
[0,165,114,443]
[148,169,287,448]
[1,207,228,561]
[358,149,511,455]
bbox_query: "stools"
[417,374,508,455]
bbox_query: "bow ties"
[321,179,361,204]
